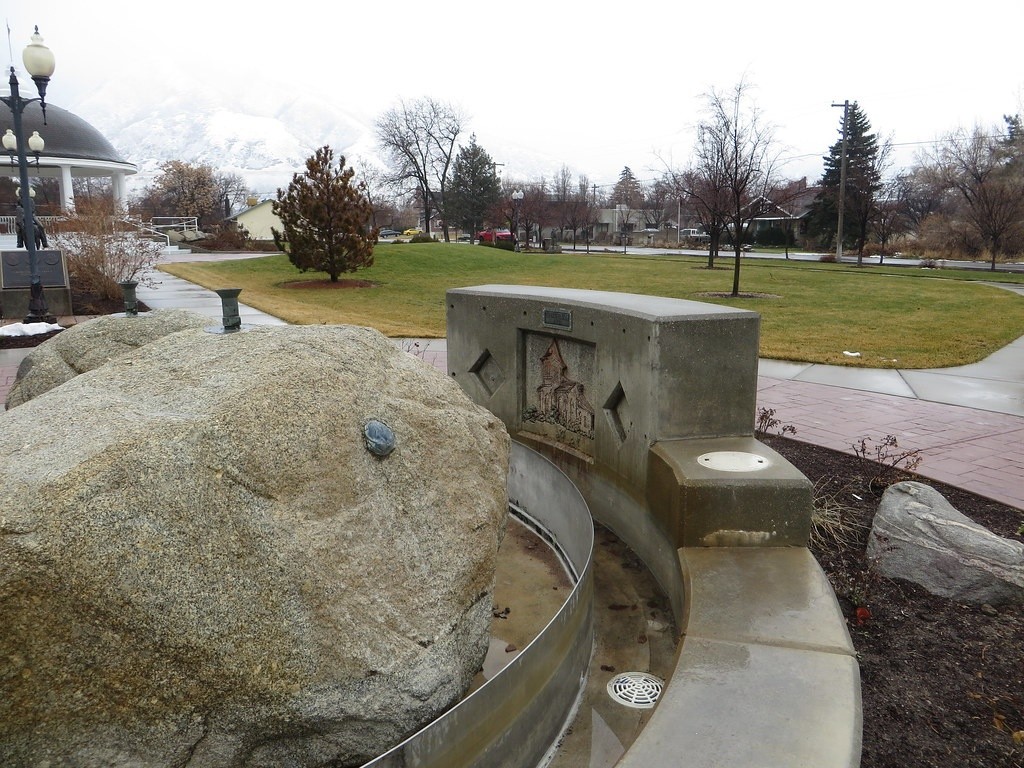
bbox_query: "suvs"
[478,225,512,243]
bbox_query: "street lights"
[0,22,57,325]
[511,189,525,252]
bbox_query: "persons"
[15,189,50,248]
[550,227,559,246]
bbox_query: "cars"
[403,228,424,236]
[379,229,402,238]
